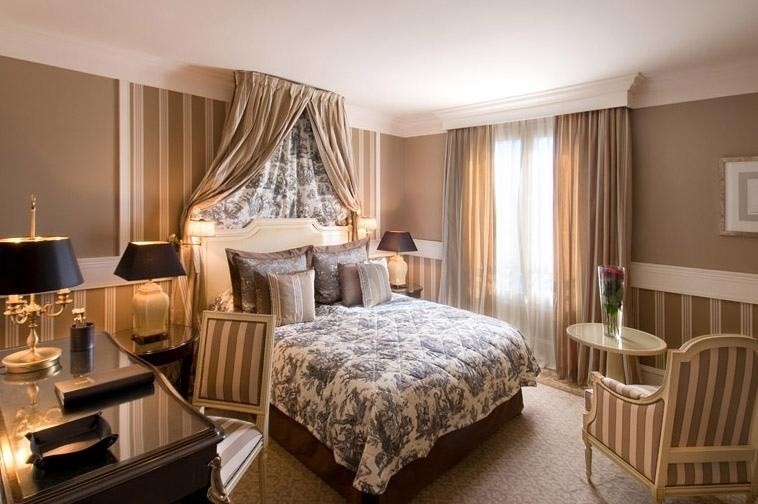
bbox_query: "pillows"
[312,246,367,304]
[335,258,366,305]
[309,236,370,306]
[224,246,316,311]
[357,257,392,307]
[265,268,317,325]
[230,253,308,316]
[252,268,308,315]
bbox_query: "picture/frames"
[717,152,758,239]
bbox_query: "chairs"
[187,309,279,504]
[577,324,757,504]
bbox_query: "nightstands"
[389,282,423,298]
[108,325,194,405]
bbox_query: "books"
[53,363,156,410]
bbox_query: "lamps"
[377,229,417,289]
[354,216,378,237]
[112,239,191,340]
[1,193,85,374]
[168,215,216,249]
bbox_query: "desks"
[564,313,670,386]
[1,329,222,504]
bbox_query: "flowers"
[597,261,626,340]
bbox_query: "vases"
[598,295,624,336]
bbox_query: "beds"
[186,212,541,502]
